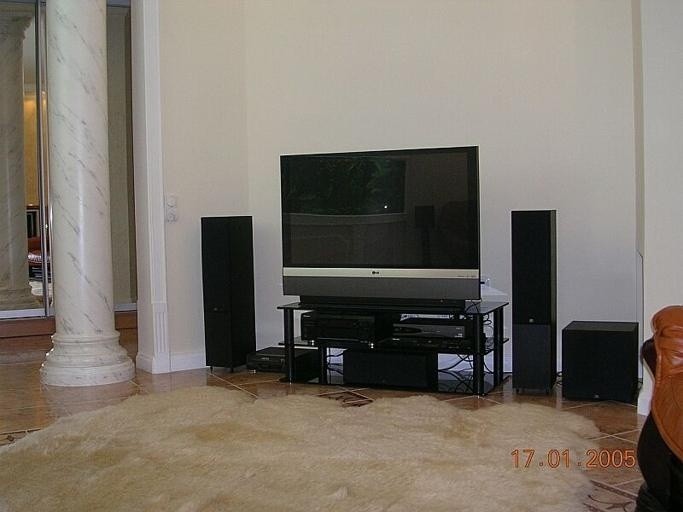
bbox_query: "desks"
[276,300,509,397]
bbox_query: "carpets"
[27,280,53,296]
[0,383,601,512]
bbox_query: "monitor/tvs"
[279,145,482,302]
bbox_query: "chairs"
[27,236,52,277]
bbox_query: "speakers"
[511,209,557,393]
[562,319,642,407]
[341,351,442,393]
[198,215,259,371]
